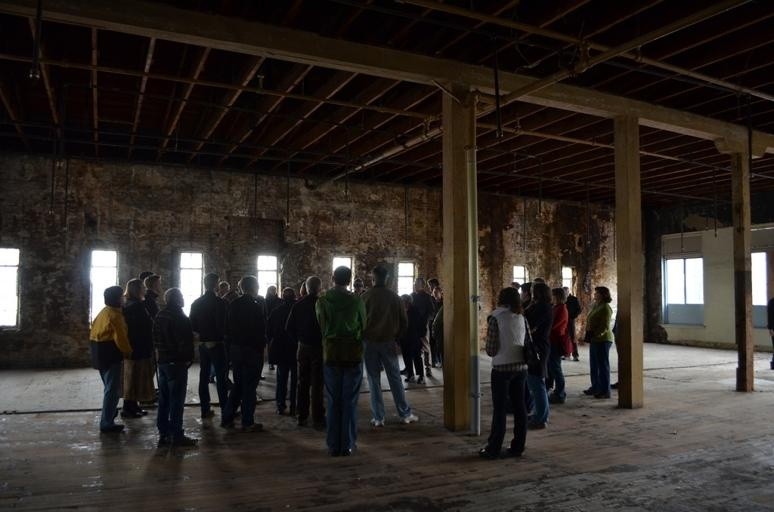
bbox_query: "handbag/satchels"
[559,334,575,355]
[522,340,540,366]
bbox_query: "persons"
[767,298,774,370]
[583,286,614,398]
[472,277,582,460]
[610,312,618,389]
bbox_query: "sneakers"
[611,383,619,389]
[583,388,611,399]
[400,368,407,374]
[111,425,125,432]
[242,422,262,433]
[417,377,425,384]
[426,367,432,376]
[405,376,415,382]
[172,436,198,447]
[369,417,385,428]
[400,413,419,425]
[133,409,148,417]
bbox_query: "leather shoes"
[478,449,494,457]
[506,446,524,453]
[329,448,351,457]
